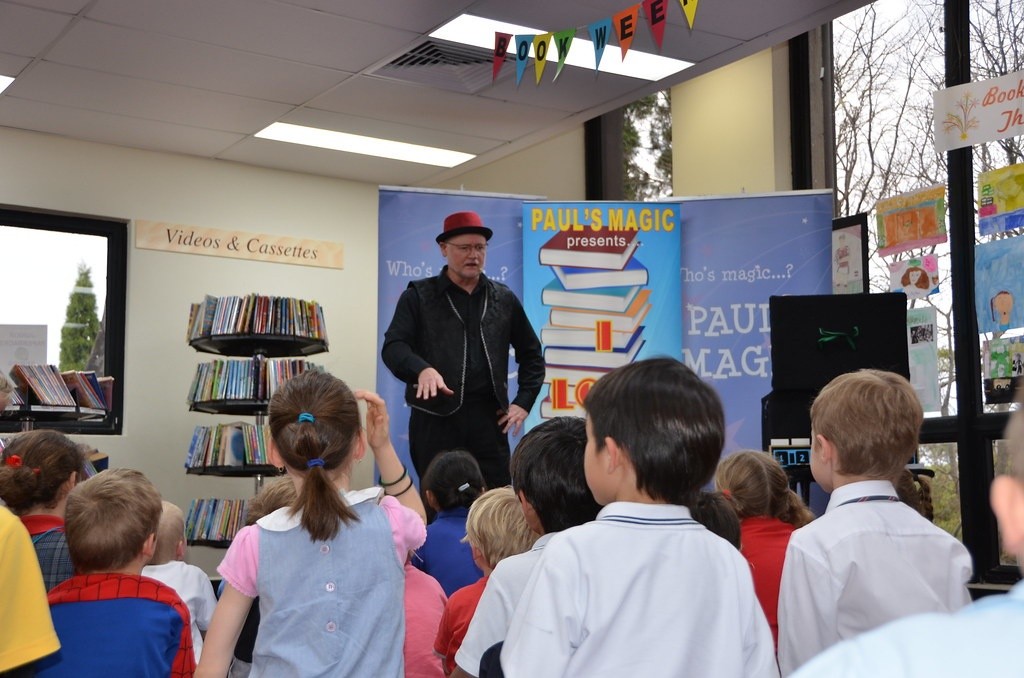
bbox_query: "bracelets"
[379,464,413,497]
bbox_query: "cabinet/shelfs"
[186,332,329,548]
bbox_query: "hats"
[435,211,493,245]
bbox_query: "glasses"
[444,241,491,252]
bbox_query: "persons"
[403,355,1024,678]
[194,370,427,678]
[1,367,261,678]
[382,212,546,527]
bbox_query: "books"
[184,293,329,540]
[7,364,113,422]
